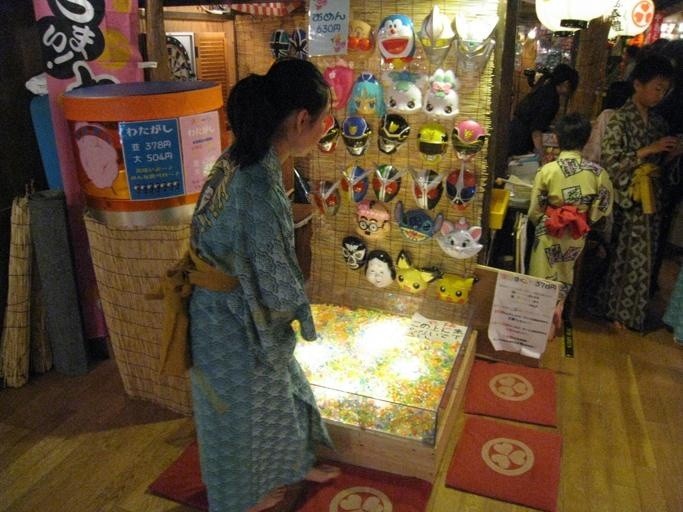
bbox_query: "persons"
[157,55,341,512]
[582,36,683,350]
[503,63,579,166]
[526,110,614,344]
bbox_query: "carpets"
[145,438,436,511]
[460,358,559,430]
[443,414,564,511]
